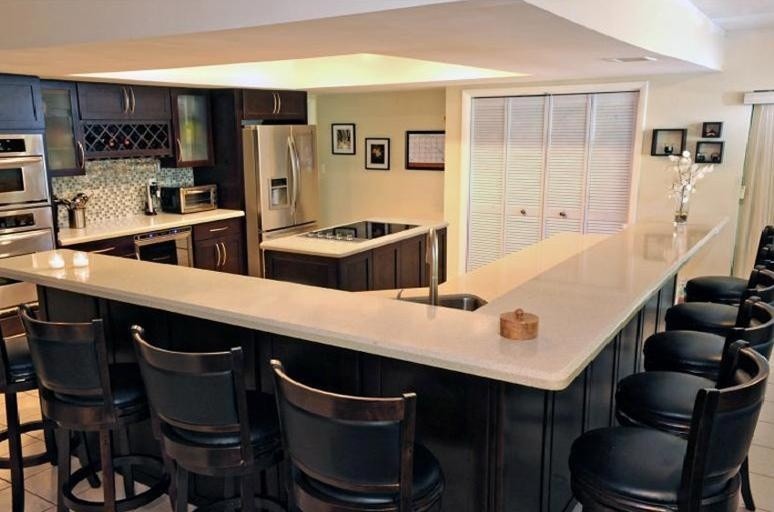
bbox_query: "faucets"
[424,230,439,306]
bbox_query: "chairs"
[271,358,446,510]
[0,332,58,511]
[16,302,169,511]
[611,294,773,511]
[568,339,773,512]
[682,226,774,303]
[130,323,281,510]
[664,264,773,337]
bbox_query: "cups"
[72,208,85,229]
[72,251,89,268]
[49,254,63,269]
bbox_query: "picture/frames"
[366,221,391,238]
[406,130,445,170]
[365,137,390,170]
[333,227,357,238]
[331,123,356,155]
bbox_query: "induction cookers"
[299,220,420,242]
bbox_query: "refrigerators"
[242,124,320,279]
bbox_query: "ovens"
[0,134,56,259]
[134,228,195,268]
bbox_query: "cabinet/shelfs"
[702,121,722,138]
[40,80,86,177]
[192,217,246,275]
[650,128,688,156]
[160,88,215,168]
[0,74,46,130]
[77,82,172,120]
[694,141,723,163]
[64,236,137,259]
[209,90,306,120]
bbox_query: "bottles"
[125,141,129,146]
[150,181,158,209]
[109,141,114,147]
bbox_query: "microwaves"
[160,183,218,216]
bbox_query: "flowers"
[664,149,713,219]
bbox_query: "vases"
[672,190,690,223]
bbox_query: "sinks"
[392,292,489,313]
